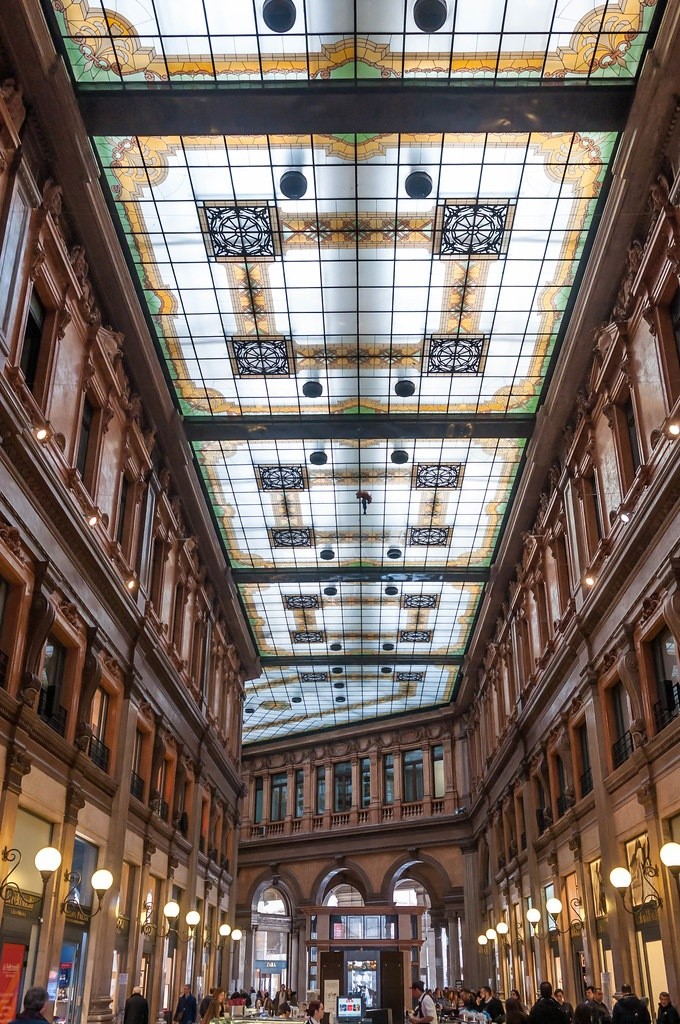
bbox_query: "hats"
[409,981,424,991]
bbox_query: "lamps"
[138,900,181,939]
[621,511,635,522]
[210,927,243,950]
[27,427,49,439]
[546,896,583,935]
[0,845,62,909]
[204,922,231,950]
[526,908,556,939]
[167,911,202,943]
[456,803,467,816]
[83,511,97,525]
[496,920,523,951]
[584,573,600,585]
[59,867,114,918]
[660,842,680,894]
[610,863,660,916]
[121,576,134,589]
[478,935,498,955]
[486,928,504,953]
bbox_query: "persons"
[255,991,275,1017]
[276,984,291,1019]
[240,989,252,1008]
[123,987,149,1024]
[200,988,225,1024]
[173,984,197,1024]
[287,989,297,1006]
[409,981,437,1024]
[7,987,51,1024]
[459,986,506,1024]
[199,986,216,1018]
[305,1001,324,1024]
[425,986,465,1017]
[279,1002,291,1018]
[506,989,531,1024]
[250,987,255,993]
[611,984,680,1024]
[577,986,612,1024]
[231,989,241,1000]
[528,982,575,1024]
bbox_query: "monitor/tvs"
[336,996,364,1022]
